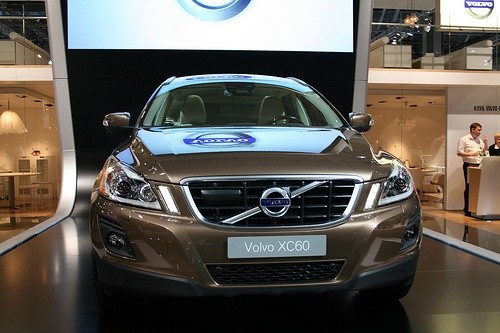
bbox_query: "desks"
[0,172,41,207]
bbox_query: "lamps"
[0,101,27,135]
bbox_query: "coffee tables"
[411,57,445,70]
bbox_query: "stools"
[33,182,52,210]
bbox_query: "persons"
[457,123,488,216]
[489,132,500,156]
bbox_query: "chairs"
[258,96,286,126]
[180,96,207,124]
[408,168,445,203]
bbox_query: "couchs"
[444,40,493,70]
[369,37,411,68]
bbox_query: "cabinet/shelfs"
[15,156,52,198]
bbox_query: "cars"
[88,73,423,318]
